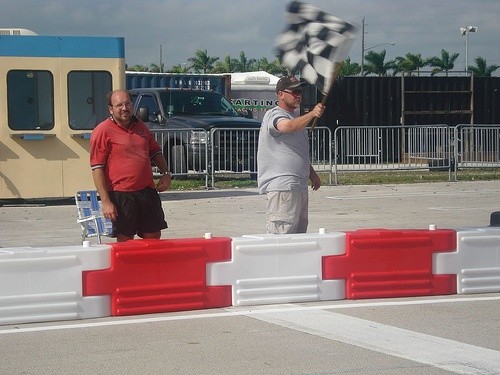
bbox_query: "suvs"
[128,87,262,179]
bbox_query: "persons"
[257,76,325,234]
[90,89,170,242]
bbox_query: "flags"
[274,1,359,95]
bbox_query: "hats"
[276,76,307,93]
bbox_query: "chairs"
[75,189,112,244]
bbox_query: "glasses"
[112,102,133,109]
[282,90,303,97]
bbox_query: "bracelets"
[163,171,171,176]
